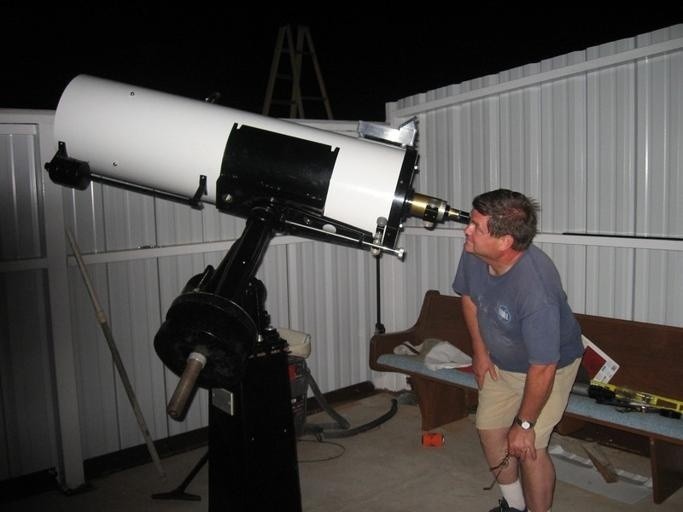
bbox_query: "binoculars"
[44,73,472,260]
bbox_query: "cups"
[422,433,446,448]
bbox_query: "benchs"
[369,289,683,506]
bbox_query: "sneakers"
[488,497,528,512]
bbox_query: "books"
[581,334,621,384]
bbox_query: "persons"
[452,189,585,512]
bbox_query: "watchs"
[513,416,535,431]
